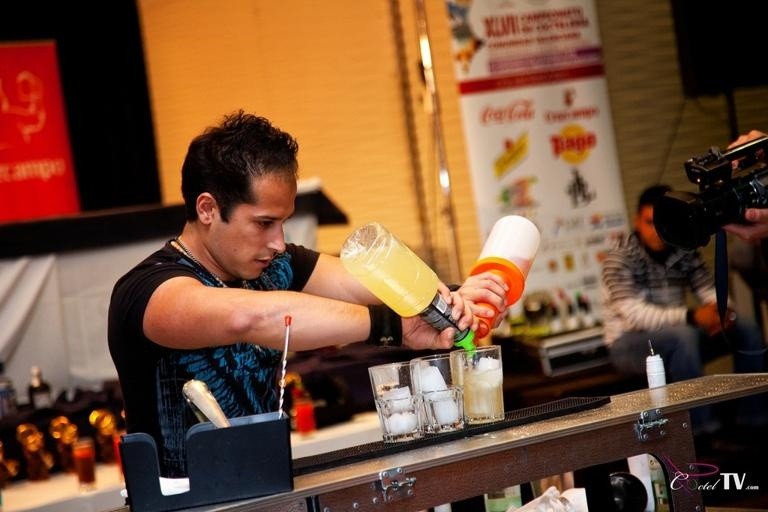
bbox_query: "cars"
[366,346,505,443]
[72,439,97,490]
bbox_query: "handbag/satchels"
[358,303,403,348]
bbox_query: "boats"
[670,0,768,99]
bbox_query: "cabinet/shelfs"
[645,354,667,388]
[342,215,544,358]
[292,392,317,439]
[1,366,119,483]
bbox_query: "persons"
[599,182,767,436]
[106,109,510,480]
[720,129,767,242]
